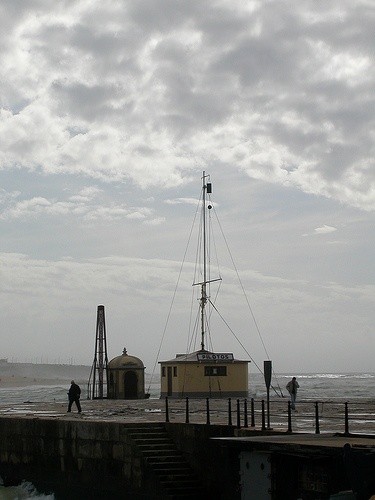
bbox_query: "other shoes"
[78,410,81,413]
[67,411,71,412]
[292,410,297,412]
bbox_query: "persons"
[286,377,299,410]
[66,380,81,414]
